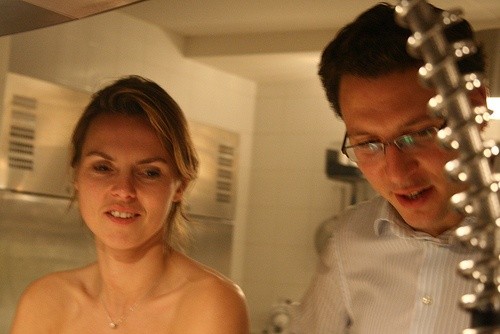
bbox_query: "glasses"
[341,119,448,163]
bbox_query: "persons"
[279,2,500,334]
[10,74,252,334]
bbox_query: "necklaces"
[99,279,159,329]
